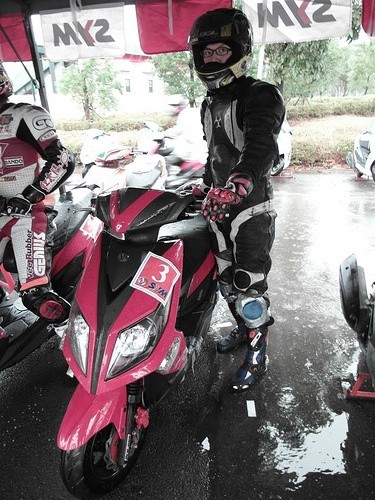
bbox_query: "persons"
[183,7,286,391]
[163,93,204,170]
[0,60,75,327]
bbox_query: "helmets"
[168,93,185,116]
[187,8,254,90]
[0,60,13,96]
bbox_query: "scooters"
[345,124,375,182]
[0,119,293,500]
[337,251,375,400]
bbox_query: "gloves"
[201,173,254,223]
[4,184,46,219]
[192,182,211,200]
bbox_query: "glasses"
[200,46,232,58]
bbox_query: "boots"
[217,301,248,353]
[230,318,274,391]
